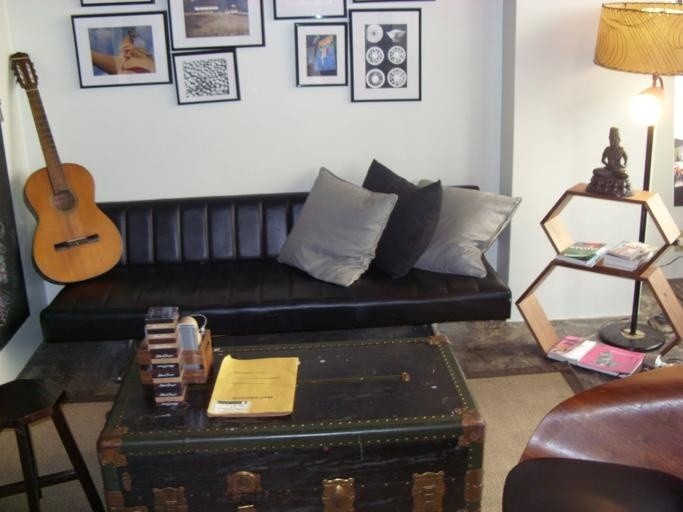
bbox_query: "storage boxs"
[96,334,486,512]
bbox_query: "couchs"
[39,183,511,341]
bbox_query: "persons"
[313,34,337,75]
[88,28,156,74]
[594,127,629,179]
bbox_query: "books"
[558,234,659,271]
[545,331,643,376]
[205,350,300,419]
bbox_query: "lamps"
[594,2,681,351]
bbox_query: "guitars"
[9,52,124,284]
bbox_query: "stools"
[1,379,102,510]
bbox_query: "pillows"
[360,160,440,290]
[275,165,397,288]
[412,179,521,277]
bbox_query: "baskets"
[138,329,213,385]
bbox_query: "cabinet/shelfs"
[514,183,681,370]
